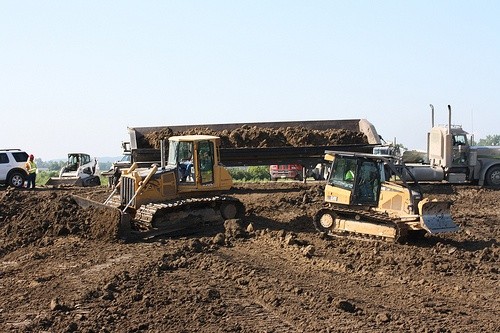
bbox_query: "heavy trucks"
[127,102,500,191]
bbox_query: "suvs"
[0,148,32,188]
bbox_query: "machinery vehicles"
[45,151,102,188]
[312,149,459,245]
[75,133,247,241]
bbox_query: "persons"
[25,154,37,190]
[177,143,199,182]
[345,162,362,184]
